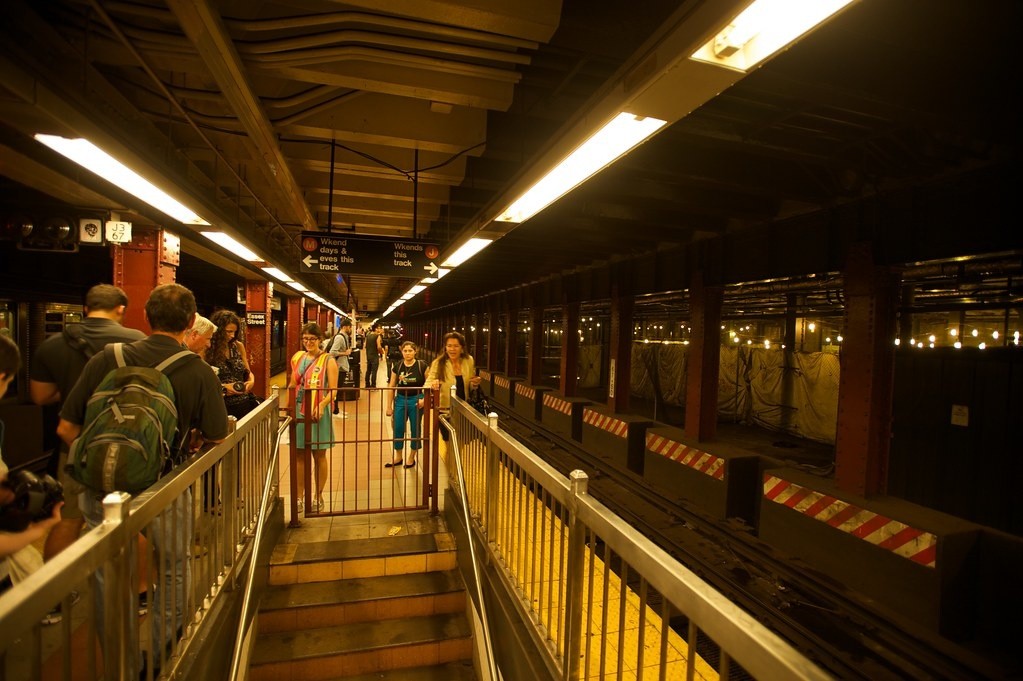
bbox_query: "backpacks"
[74,342,196,496]
[387,339,402,359]
[325,333,348,361]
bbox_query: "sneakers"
[297,498,304,512]
[311,499,324,512]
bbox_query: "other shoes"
[203,504,223,516]
[385,459,403,467]
[370,386,380,392]
[190,544,207,558]
[72,591,81,607]
[42,602,62,624]
[139,583,156,614]
[237,497,244,508]
[403,460,415,468]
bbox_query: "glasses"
[301,337,319,343]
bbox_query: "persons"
[0,334,63,680]
[385,330,403,382]
[325,319,352,419]
[57,284,229,681]
[182,311,218,355]
[286,324,339,513]
[30,284,148,624]
[203,310,259,516]
[364,323,384,390]
[385,342,430,469]
[357,324,364,336]
[421,332,482,479]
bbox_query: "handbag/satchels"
[466,384,491,419]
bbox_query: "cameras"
[233,381,245,393]
[0,470,64,534]
